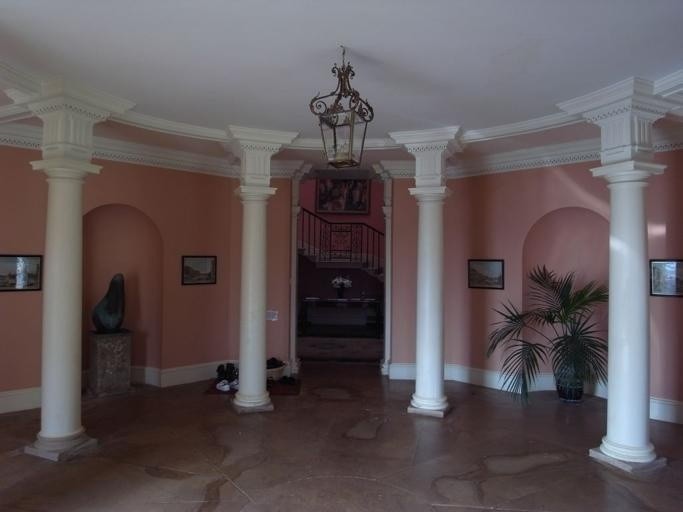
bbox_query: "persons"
[320,179,368,211]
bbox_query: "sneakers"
[216,379,239,391]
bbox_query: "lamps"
[309,45,374,169]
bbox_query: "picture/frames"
[468,258,504,290]
[648,258,682,298]
[0,254,41,292]
[180,255,216,285]
[314,177,371,216]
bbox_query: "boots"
[216,363,234,383]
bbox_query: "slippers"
[279,376,296,384]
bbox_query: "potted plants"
[486,265,608,404]
[266,356,287,380]
